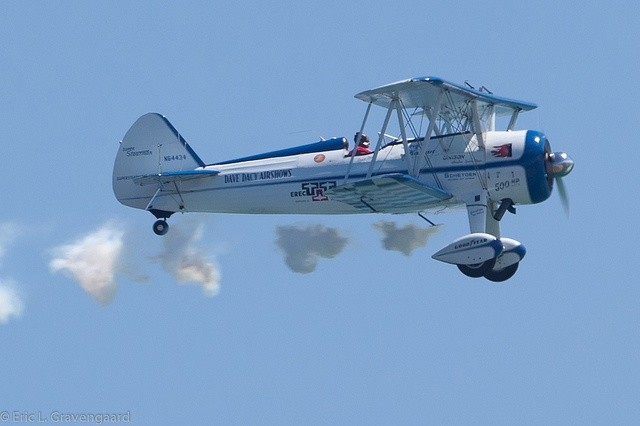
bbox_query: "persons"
[348,131,374,156]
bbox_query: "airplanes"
[113,77,574,282]
